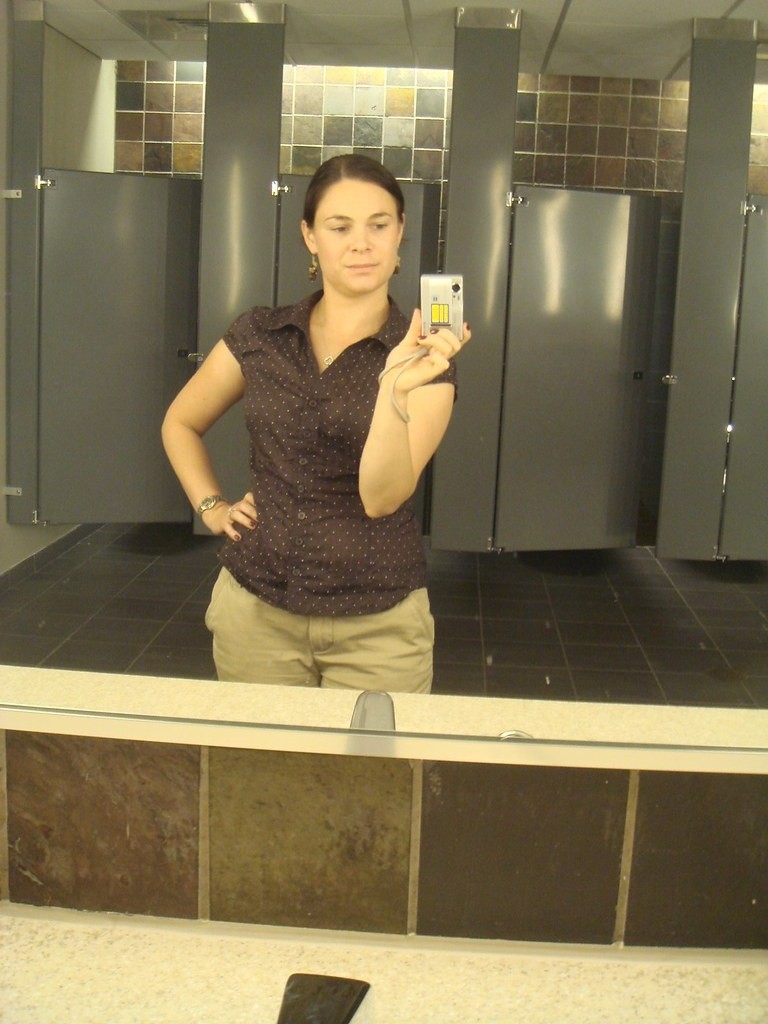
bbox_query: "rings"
[228,509,236,517]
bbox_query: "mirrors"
[0,0,768,754]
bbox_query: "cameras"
[420,275,463,343]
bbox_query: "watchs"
[198,494,228,516]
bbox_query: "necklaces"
[316,302,358,366]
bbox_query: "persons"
[159,155,472,693]
